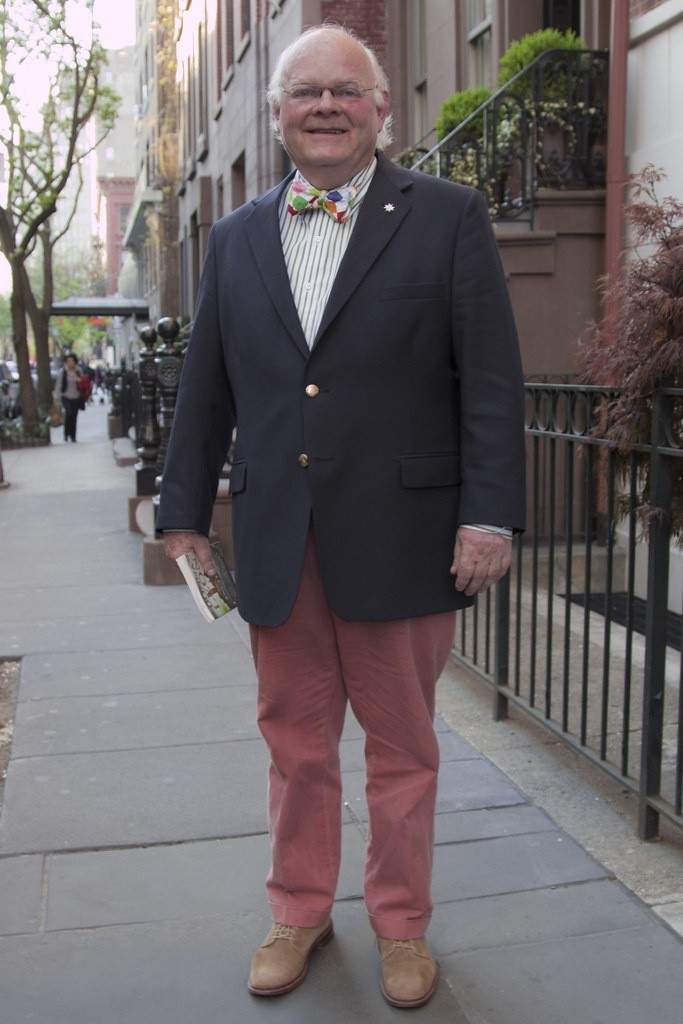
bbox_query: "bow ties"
[287,182,357,223]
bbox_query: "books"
[174,540,238,622]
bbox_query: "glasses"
[280,85,375,104]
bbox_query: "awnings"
[50,295,150,316]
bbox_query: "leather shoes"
[378,937,437,1007]
[248,916,334,995]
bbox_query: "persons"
[78,357,129,410]
[55,353,85,443]
[149,24,526,1009]
